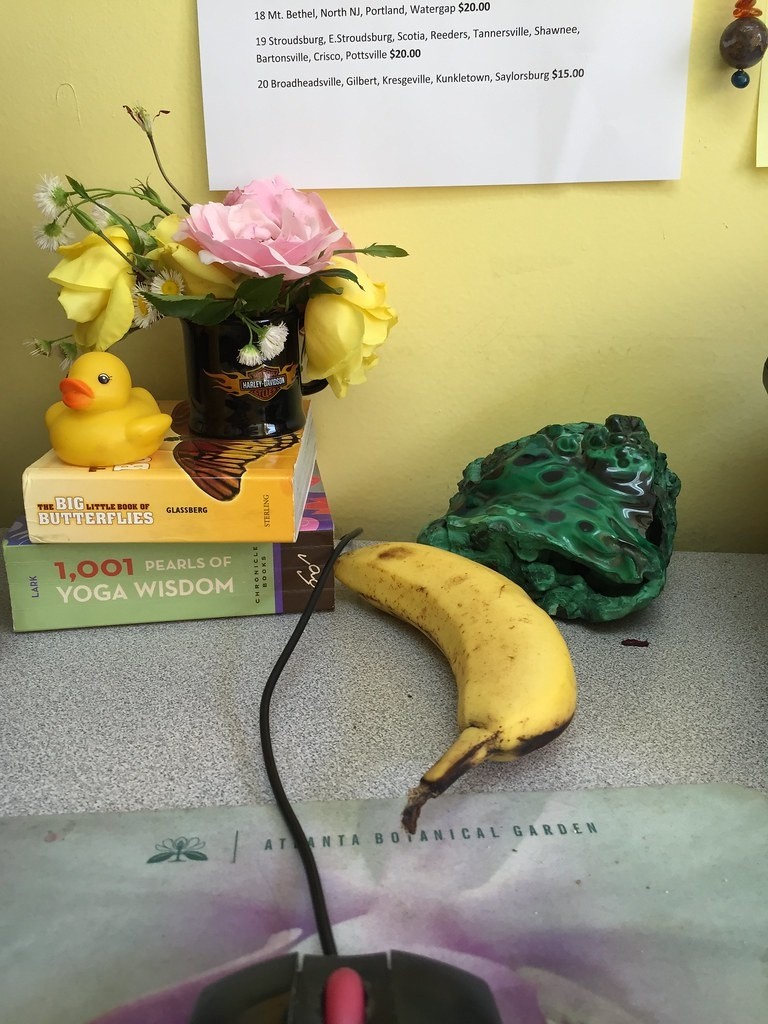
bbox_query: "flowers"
[32,104,415,401]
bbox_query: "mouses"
[192,948,503,1024]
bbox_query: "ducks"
[44,351,173,467]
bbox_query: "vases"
[178,306,308,442]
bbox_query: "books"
[2,459,335,633]
[20,398,319,544]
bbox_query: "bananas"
[330,540,579,836]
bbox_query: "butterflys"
[163,400,301,501]
